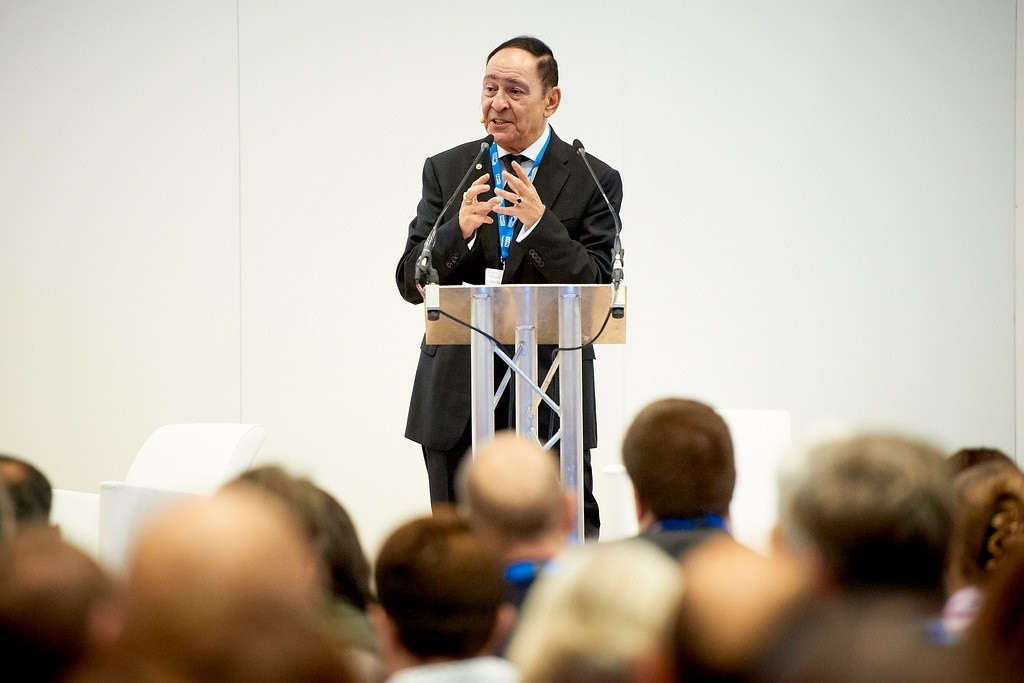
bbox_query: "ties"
[499,154,532,224]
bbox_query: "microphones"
[415,134,495,280]
[573,139,621,281]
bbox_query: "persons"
[1,399,1024,683]
[395,34,623,544]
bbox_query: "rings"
[515,197,523,205]
[463,192,473,202]
